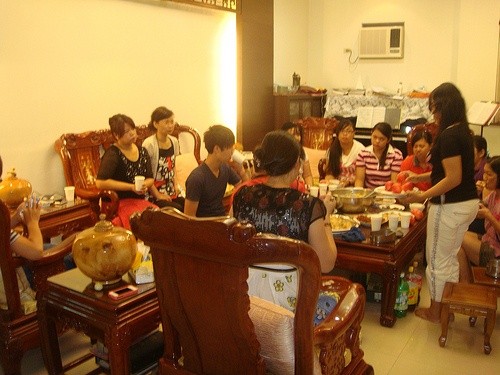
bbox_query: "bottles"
[406,260,422,307]
[404,266,418,313]
[394,272,409,318]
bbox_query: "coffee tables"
[12,196,100,300]
[35,264,163,375]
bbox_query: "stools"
[438,281,497,355]
[471,266,500,295]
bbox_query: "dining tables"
[331,203,428,328]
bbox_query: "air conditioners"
[357,25,404,59]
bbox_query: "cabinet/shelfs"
[273,92,328,132]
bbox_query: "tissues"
[127,238,155,284]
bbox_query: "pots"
[331,189,376,213]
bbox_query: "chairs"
[0,198,84,375]
[406,122,474,156]
[128,205,376,374]
[294,116,338,151]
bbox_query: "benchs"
[54,120,203,221]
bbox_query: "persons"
[142,107,186,216]
[355,120,404,191]
[462,135,491,236]
[398,130,434,196]
[455,156,500,288]
[96,114,161,236]
[0,154,78,314]
[323,119,364,189]
[396,82,478,325]
[233,123,341,328]
[182,125,243,223]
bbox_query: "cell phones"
[20,190,44,216]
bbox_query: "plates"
[374,185,420,197]
[330,197,406,233]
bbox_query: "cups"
[231,149,245,165]
[400,211,412,229]
[134,176,145,191]
[64,186,75,201]
[308,180,340,198]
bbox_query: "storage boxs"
[355,106,402,131]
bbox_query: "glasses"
[339,129,355,135]
